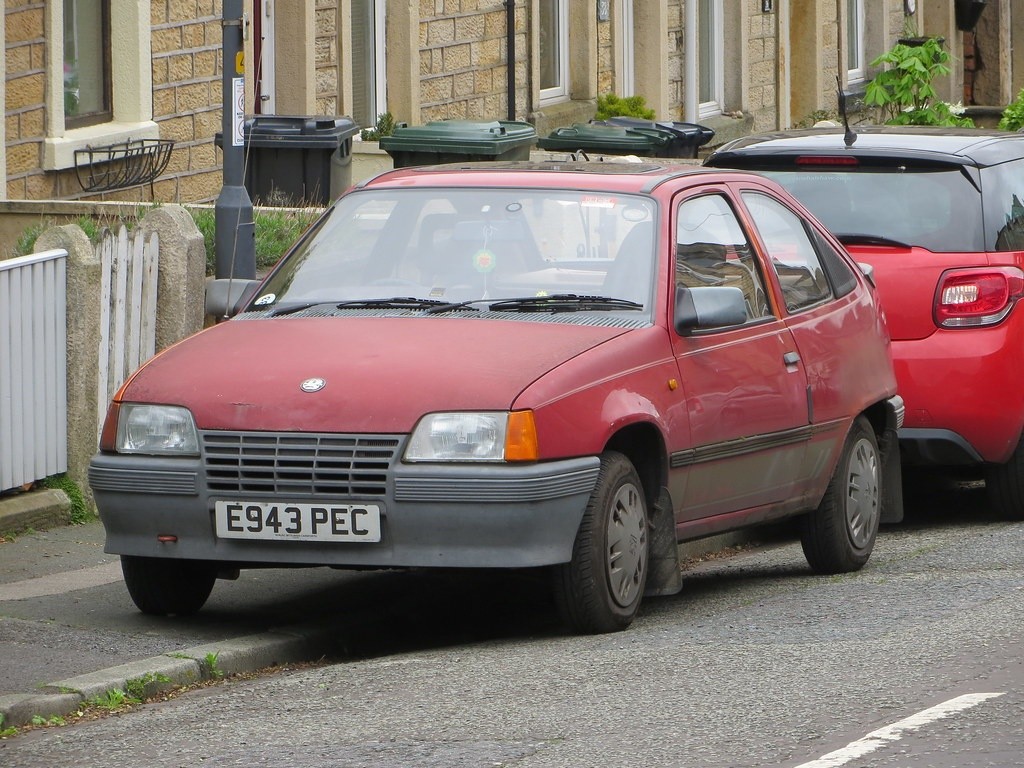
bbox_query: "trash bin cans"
[536,116,718,159]
[214,113,361,208]
[377,117,538,171]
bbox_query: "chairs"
[600,221,728,306]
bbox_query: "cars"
[703,123,1023,522]
[87,150,905,636]
